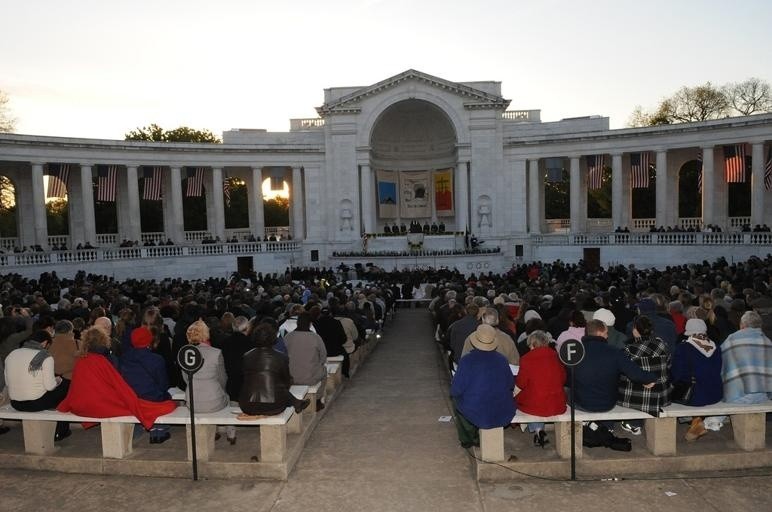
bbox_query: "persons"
[231,236,238,243]
[471,234,478,244]
[650,225,657,233]
[149,240,155,245]
[159,240,165,245]
[219,317,250,401]
[257,236,261,241]
[284,311,326,411]
[520,259,609,318]
[425,280,440,343]
[626,298,678,347]
[512,334,567,449]
[278,262,400,309]
[270,234,276,241]
[83,242,93,249]
[751,299,772,339]
[678,255,751,318]
[711,306,738,345]
[667,226,673,232]
[0,309,34,363]
[119,239,139,247]
[680,225,686,232]
[672,319,722,442]
[280,234,286,241]
[1,245,46,264]
[0,358,10,436]
[94,316,122,355]
[411,281,424,308]
[402,280,413,307]
[449,303,482,373]
[618,315,671,436]
[742,224,751,232]
[709,224,716,232]
[263,235,268,241]
[0,270,114,321]
[226,237,230,243]
[239,323,310,416]
[248,234,256,242]
[309,307,320,323]
[4,331,72,442]
[441,303,464,353]
[622,226,629,232]
[688,225,695,232]
[657,226,665,233]
[215,236,221,244]
[315,308,350,379]
[441,263,520,310]
[669,301,687,335]
[761,224,770,232]
[32,316,55,340]
[401,266,459,276]
[392,222,399,232]
[278,304,316,342]
[401,223,406,232]
[59,242,68,250]
[114,270,277,334]
[695,225,701,232]
[113,327,186,443]
[450,325,516,448]
[208,236,215,243]
[77,243,83,250]
[439,222,445,231]
[431,222,438,232]
[609,263,682,297]
[753,224,761,232]
[361,302,375,329]
[703,225,712,232]
[47,319,81,388]
[166,238,173,245]
[202,236,208,244]
[593,308,628,349]
[144,239,149,245]
[751,253,772,304]
[673,225,679,232]
[703,311,772,431]
[609,291,636,333]
[384,222,390,233]
[181,321,236,441]
[375,309,390,326]
[714,225,721,232]
[615,226,623,232]
[52,243,60,251]
[461,308,520,376]
[518,318,556,355]
[332,304,358,354]
[564,320,657,435]
[558,311,587,354]
[57,327,177,430]
[423,221,430,233]
[341,301,366,345]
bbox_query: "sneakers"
[621,420,642,435]
[684,418,705,440]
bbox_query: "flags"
[697,148,702,194]
[97,164,117,201]
[187,166,203,197]
[224,169,230,208]
[142,166,162,200]
[587,154,603,191]
[47,163,70,198]
[764,142,772,190]
[723,143,745,183]
[631,152,649,188]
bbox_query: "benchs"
[396,299,432,309]
[1,403,295,462]
[480,401,772,461]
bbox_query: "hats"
[593,308,615,326]
[684,318,708,336]
[469,324,500,351]
[130,328,153,349]
[634,300,655,313]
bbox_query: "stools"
[327,355,344,384]
[325,364,340,394]
[304,382,322,413]
[288,385,308,433]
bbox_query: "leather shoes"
[539,430,547,449]
[156,432,170,443]
[344,375,349,380]
[0,425,10,435]
[227,436,236,443]
[316,400,325,411]
[153,434,156,444]
[534,436,550,445]
[214,433,221,440]
[296,398,311,413]
[55,429,72,440]
